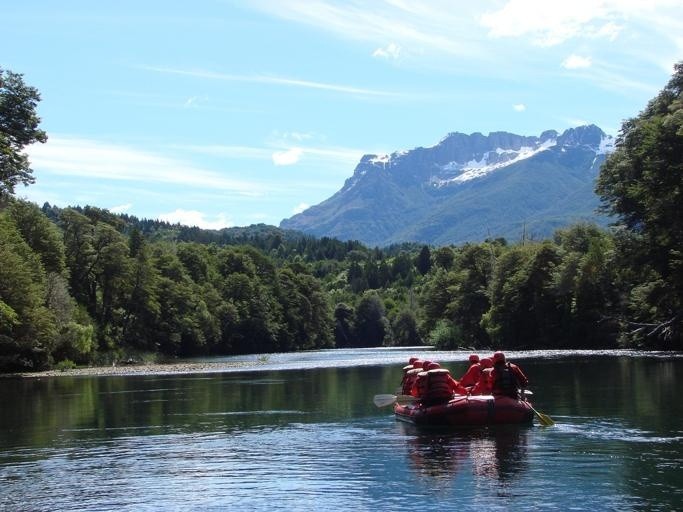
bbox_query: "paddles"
[373,394,422,408]
[517,393,554,425]
[518,389,533,395]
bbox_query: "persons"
[399,350,529,409]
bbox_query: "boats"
[392,385,535,428]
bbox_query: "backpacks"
[495,362,518,388]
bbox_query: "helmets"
[470,352,505,368]
[409,357,440,371]
